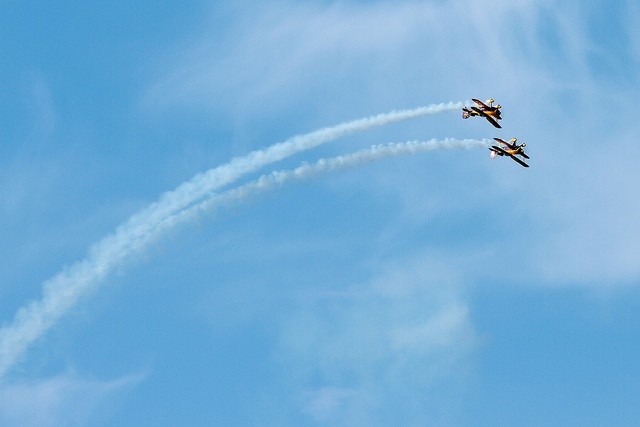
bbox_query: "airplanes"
[491,135,530,169]
[459,97,505,131]
